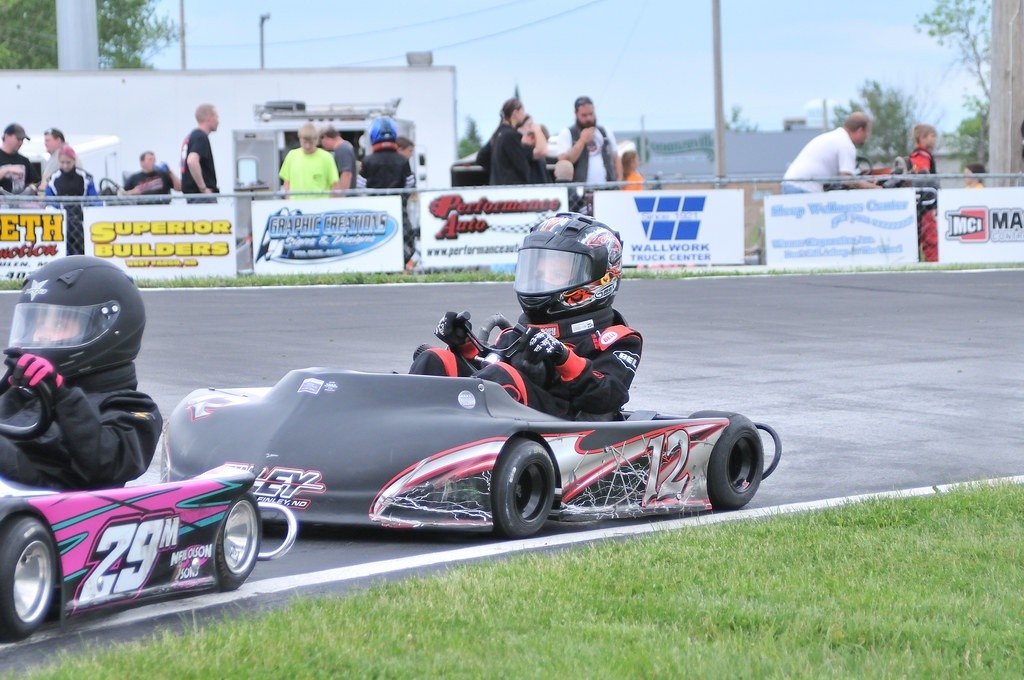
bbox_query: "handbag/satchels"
[476,126,499,171]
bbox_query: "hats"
[4,123,31,141]
[58,145,76,160]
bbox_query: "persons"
[406,212,642,421]
[554,96,623,190]
[909,123,942,262]
[962,163,986,189]
[620,150,646,191]
[278,116,417,264]
[487,98,552,186]
[181,104,219,204]
[0,123,104,256]
[120,150,182,205]
[0,255,163,494]
[781,111,882,195]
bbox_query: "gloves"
[518,326,569,368]
[435,311,472,347]
[4,347,72,409]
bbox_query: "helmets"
[368,116,398,142]
[513,212,624,324]
[3,254,147,380]
